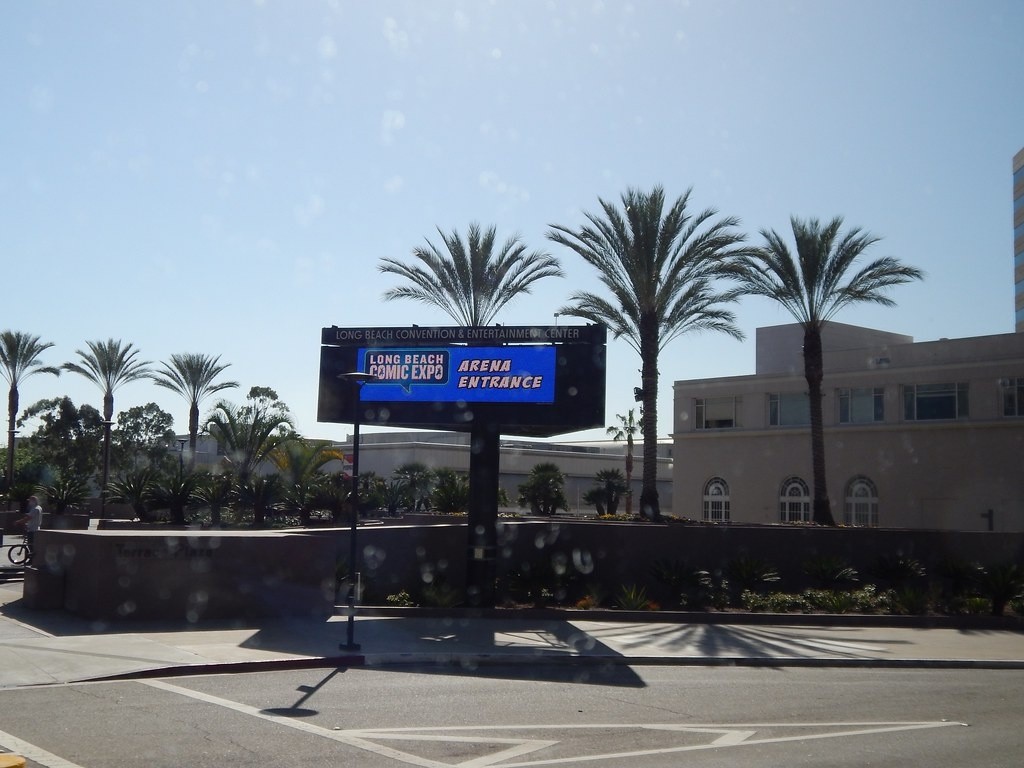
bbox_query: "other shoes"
[25,559,32,566]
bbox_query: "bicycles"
[7,526,32,565]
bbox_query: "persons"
[15,496,42,568]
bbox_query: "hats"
[26,496,38,502]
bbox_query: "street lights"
[100,420,116,519]
[176,437,189,491]
[339,372,375,666]
[6,429,21,510]
